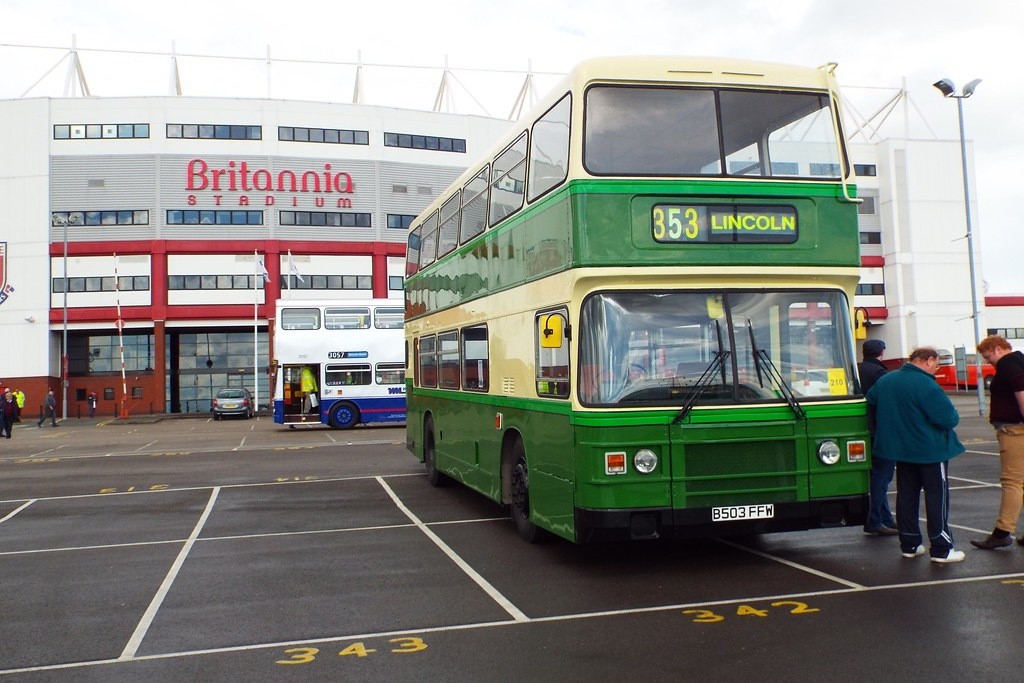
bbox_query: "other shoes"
[5,435,10,438]
[37,422,41,428]
[970,533,1013,550]
[52,424,59,427]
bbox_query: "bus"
[405,56,875,549]
[273,298,489,428]
[933,296,1024,389]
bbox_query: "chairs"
[541,365,568,395]
[580,364,615,404]
[420,365,436,387]
[466,359,488,389]
[437,363,460,388]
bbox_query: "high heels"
[873,525,899,535]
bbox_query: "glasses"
[934,360,940,370]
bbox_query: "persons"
[0,391,19,438]
[969,336,1024,548]
[12,388,25,417]
[37,390,59,427]
[88,392,97,416]
[859,340,899,534]
[301,365,318,415]
[865,348,965,563]
[0,387,10,437]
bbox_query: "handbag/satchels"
[309,392,319,407]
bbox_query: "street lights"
[49,212,78,418]
[935,76,992,415]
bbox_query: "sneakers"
[902,545,925,557]
[930,548,965,563]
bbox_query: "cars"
[213,387,255,420]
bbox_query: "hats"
[863,340,886,354]
